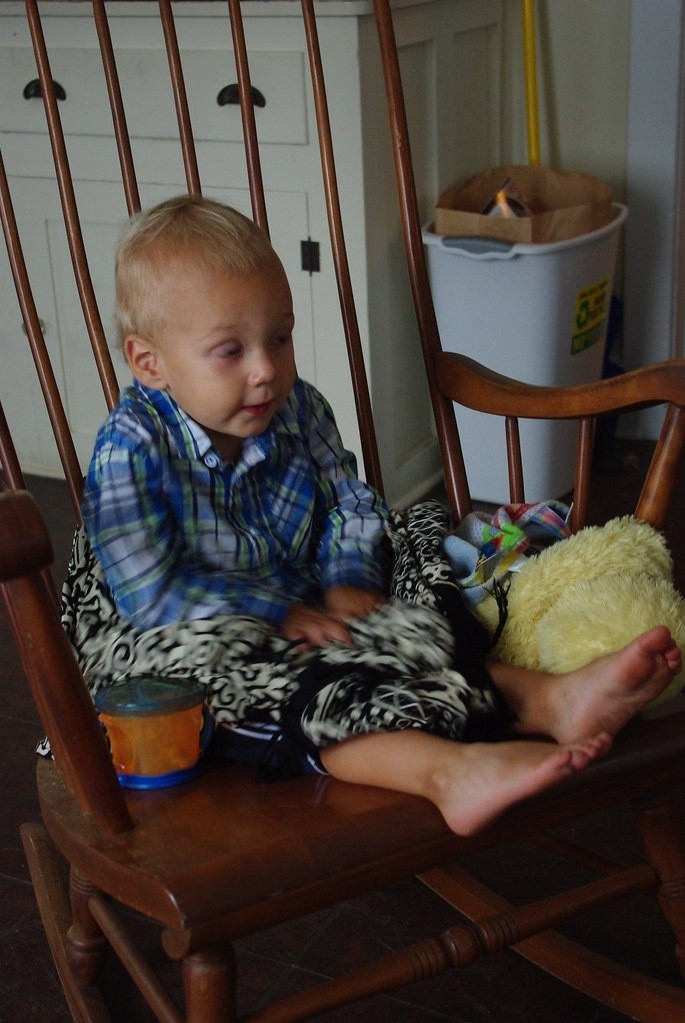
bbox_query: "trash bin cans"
[415,197,634,511]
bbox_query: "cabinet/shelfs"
[0,0,507,525]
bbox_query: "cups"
[96,673,216,790]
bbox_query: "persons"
[36,195,684,834]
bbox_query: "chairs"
[0,0,685,1023]
